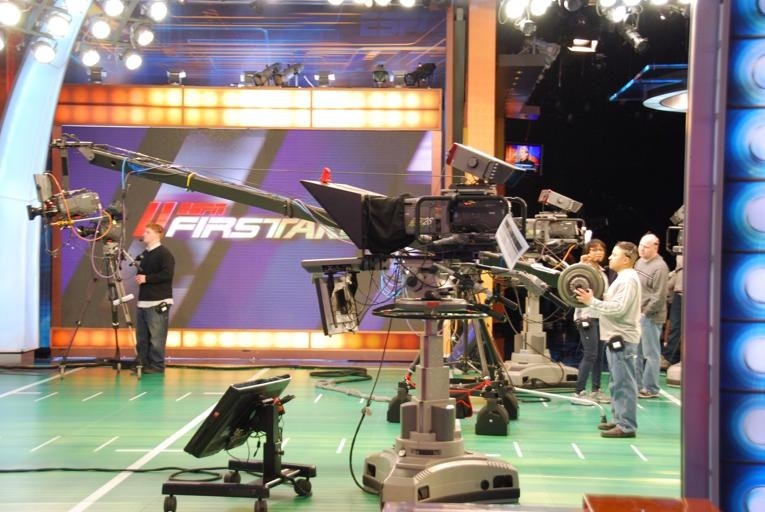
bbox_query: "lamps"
[595,0,692,54]
[81,1,167,70]
[496,0,587,35]
[0,1,72,63]
[609,64,688,114]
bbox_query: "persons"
[125,223,175,375]
[513,147,535,172]
[570,238,613,406]
[632,232,670,398]
[573,240,643,439]
[501,214,684,369]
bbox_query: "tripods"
[57,239,142,379]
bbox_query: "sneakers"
[572,389,600,405]
[598,422,616,430]
[601,424,636,437]
[590,388,611,403]
[142,366,164,373]
[130,362,150,370]
[639,388,659,398]
